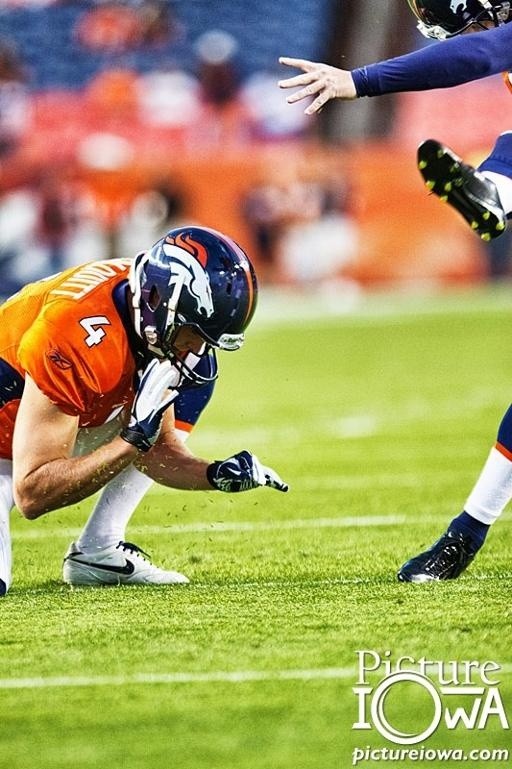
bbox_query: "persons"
[2,224,290,599]
[2,1,359,322]
[278,0,510,581]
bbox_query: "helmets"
[407,0,511,40]
[126,225,258,360]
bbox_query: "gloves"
[120,357,180,454]
[205,450,289,493]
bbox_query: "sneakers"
[60,541,191,585]
[397,519,486,584]
[415,139,508,243]
